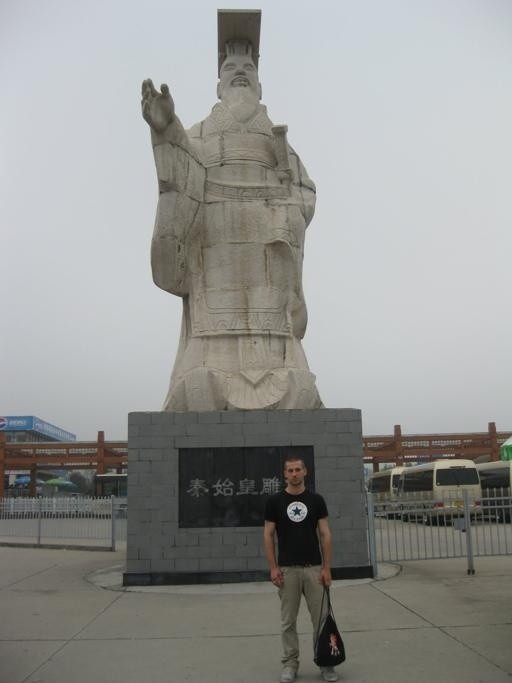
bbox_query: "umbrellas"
[14,475,44,483]
[44,477,79,490]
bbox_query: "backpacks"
[314,586,345,667]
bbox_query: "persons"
[262,454,341,683]
[8,482,42,497]
[137,6,328,409]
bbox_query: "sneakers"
[319,666,338,681]
[281,666,298,683]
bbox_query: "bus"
[365,456,512,526]
[92,471,129,520]
